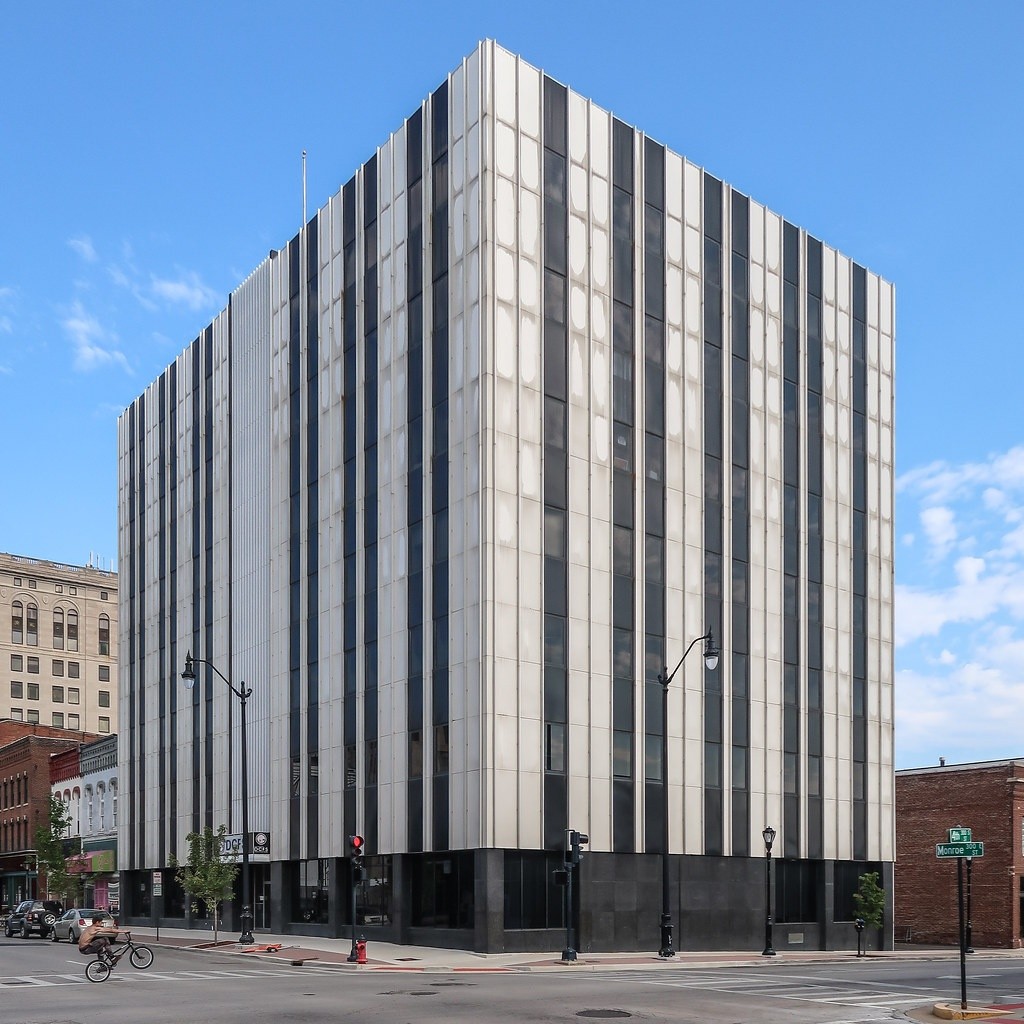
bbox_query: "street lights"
[658,626,720,957]
[760,824,779,955]
[180,646,255,944]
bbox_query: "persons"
[76,913,130,971]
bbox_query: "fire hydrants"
[356,934,367,964]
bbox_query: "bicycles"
[85,931,154,983]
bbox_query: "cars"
[50,908,119,944]
[356,905,389,927]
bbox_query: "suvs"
[4,899,65,939]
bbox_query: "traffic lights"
[570,831,589,846]
[552,870,568,886]
[348,834,364,866]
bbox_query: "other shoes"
[98,966,112,970]
[112,955,121,965]
